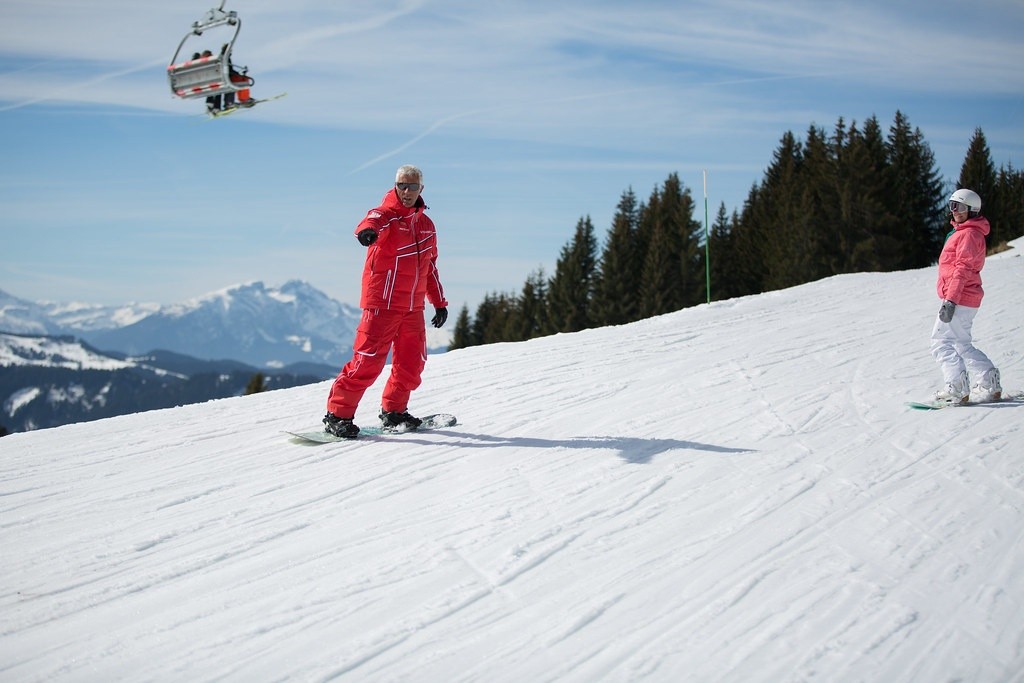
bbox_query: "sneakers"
[967,367,1002,401]
[935,371,970,406]
[322,412,360,437]
[378,408,422,430]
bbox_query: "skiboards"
[204,92,288,119]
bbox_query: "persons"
[323,164,448,438]
[926,189,1002,406]
[192,44,255,116]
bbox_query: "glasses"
[949,201,969,213]
[396,182,420,191]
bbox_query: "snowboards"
[903,388,1023,412]
[279,412,458,444]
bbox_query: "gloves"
[431,308,448,328]
[938,301,956,323]
[357,229,376,246]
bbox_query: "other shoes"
[223,102,239,109]
[207,102,220,114]
[241,97,255,106]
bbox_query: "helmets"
[949,189,981,212]
